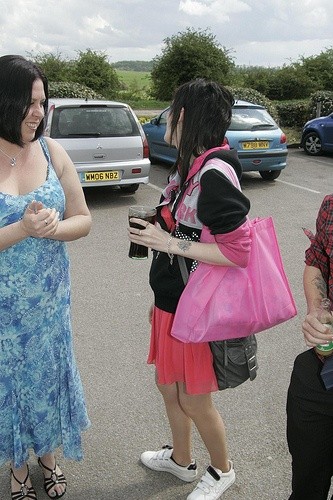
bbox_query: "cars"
[141,99,289,179]
[45,97,152,195]
[298,111,333,156]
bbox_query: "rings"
[138,230,142,235]
[44,219,49,226]
[28,205,34,212]
[144,221,149,227]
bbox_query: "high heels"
[10,461,37,500]
[38,456,67,498]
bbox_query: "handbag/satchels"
[210,334,260,391]
[170,159,297,343]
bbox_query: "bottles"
[315,297,333,356]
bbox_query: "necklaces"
[0,147,24,167]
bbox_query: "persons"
[287,193,333,500]
[0,55,93,500]
[126,78,252,499]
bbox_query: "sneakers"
[187,460,236,500]
[140,448,197,483]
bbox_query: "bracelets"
[167,236,175,259]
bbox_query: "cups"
[128,206,157,260]
